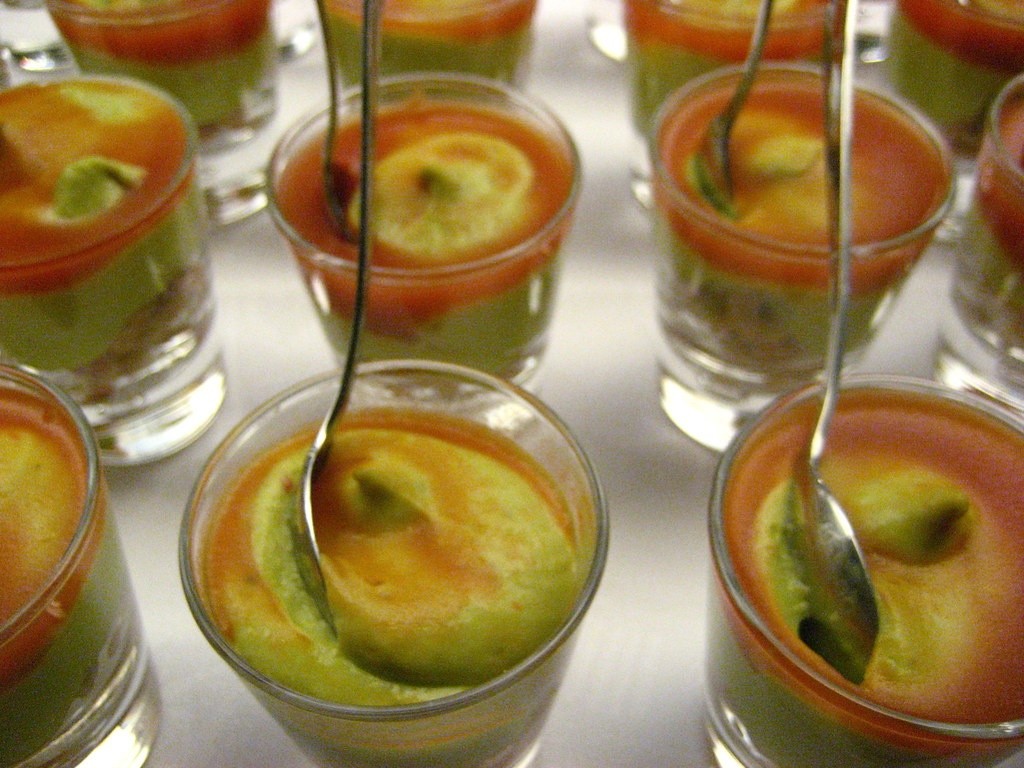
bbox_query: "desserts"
[0,0,1023,768]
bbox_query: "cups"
[1,71,230,469]
[262,70,583,433]
[316,0,540,117]
[177,357,610,767]
[1,358,167,768]
[691,373,1023,767]
[640,59,956,453]
[928,77,1022,416]
[620,1,851,205]
[48,1,290,229]
[929,75,1023,436]
[876,0,1024,243]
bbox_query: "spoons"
[294,0,377,639]
[315,1,359,244]
[785,0,883,687]
[692,0,776,218]
